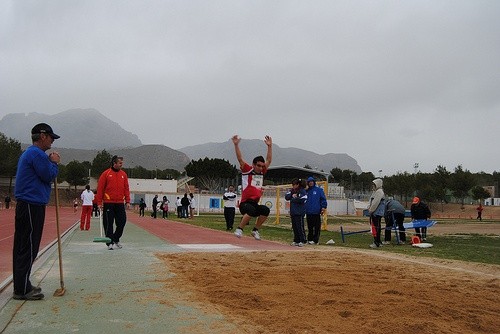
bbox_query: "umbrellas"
[177,177,195,192]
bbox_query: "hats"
[31,122,61,140]
[412,197,420,204]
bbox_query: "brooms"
[92,208,111,243]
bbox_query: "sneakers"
[251,230,261,241]
[113,241,123,248]
[108,244,114,250]
[233,228,243,238]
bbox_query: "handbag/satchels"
[369,215,377,237]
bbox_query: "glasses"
[292,183,296,185]
[256,164,265,169]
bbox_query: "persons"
[96,155,131,250]
[382,199,407,245]
[12,123,61,300]
[223,186,237,231]
[176,192,196,220]
[411,197,431,242]
[79,185,95,231]
[231,135,272,241]
[366,178,385,248]
[159,196,170,220]
[150,195,160,219]
[72,189,104,219]
[476,203,483,222]
[285,177,327,246]
[4,195,11,209]
[139,198,145,217]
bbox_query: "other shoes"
[370,243,383,248]
[226,227,233,230]
[395,240,406,245]
[306,241,315,244]
[382,241,390,245]
[291,242,300,246]
[13,285,43,301]
[299,242,303,246]
[423,238,427,241]
[420,238,423,241]
